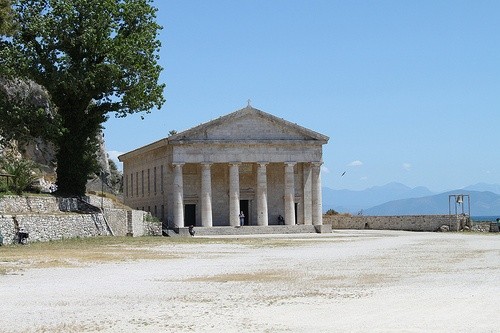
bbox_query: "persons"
[239,211,245,226]
